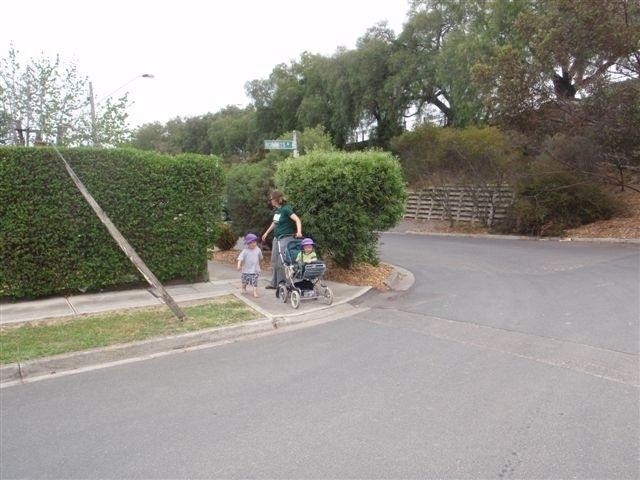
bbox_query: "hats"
[244,233,260,245]
[300,237,316,250]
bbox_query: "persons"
[235,233,265,299]
[293,236,320,265]
[260,188,303,290]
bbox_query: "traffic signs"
[264,139,297,151]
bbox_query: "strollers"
[278,233,334,309]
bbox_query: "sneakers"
[265,284,276,290]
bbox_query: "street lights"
[89,73,155,122]
